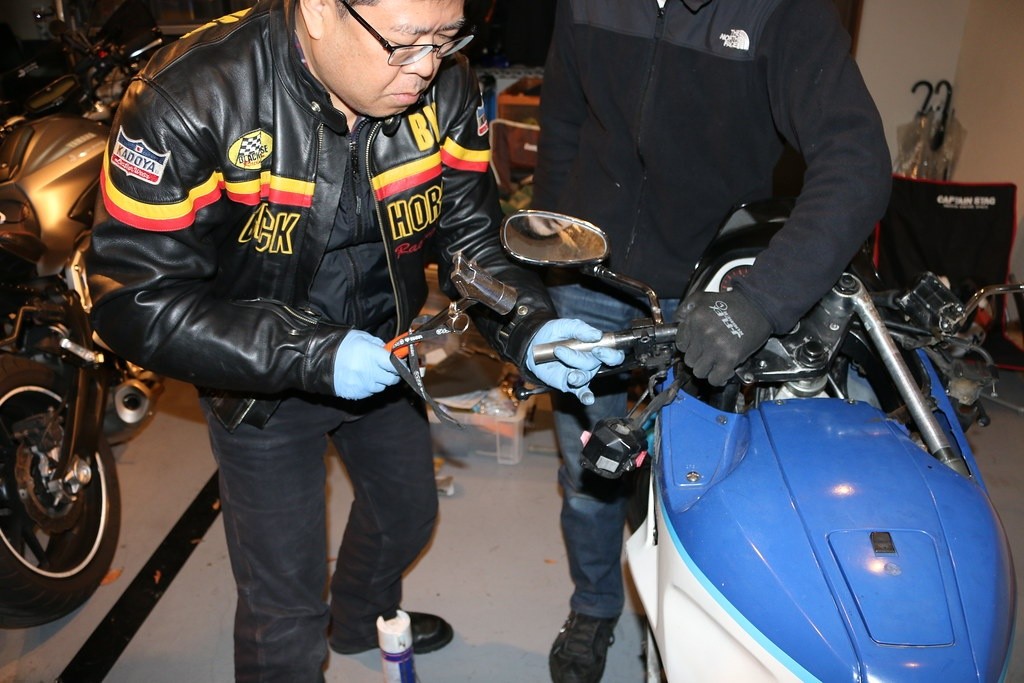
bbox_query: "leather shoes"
[329,610,454,654]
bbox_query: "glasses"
[340,0,474,67]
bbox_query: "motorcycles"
[1,0,169,621]
[497,207,1024,683]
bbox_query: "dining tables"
[870,173,1024,417]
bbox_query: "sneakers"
[550,613,618,683]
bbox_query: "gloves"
[526,319,626,406]
[334,327,410,399]
[672,290,773,387]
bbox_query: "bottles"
[376,610,416,683]
[479,70,496,123]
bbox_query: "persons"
[529,0,893,683]
[90,0,625,683]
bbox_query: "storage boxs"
[424,387,527,464]
